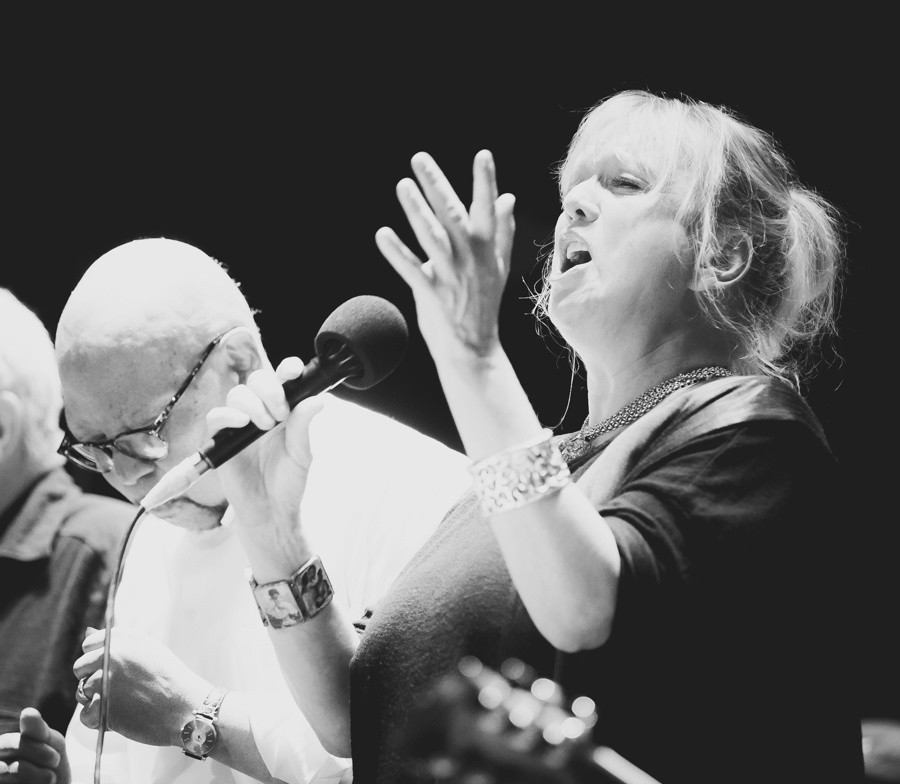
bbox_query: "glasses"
[58,326,236,473]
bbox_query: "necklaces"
[554,366,738,465]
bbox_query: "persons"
[203,90,842,784]
[0,287,140,731]
[15,236,473,784]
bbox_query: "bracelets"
[247,550,336,628]
[469,426,574,522]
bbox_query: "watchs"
[178,688,227,762]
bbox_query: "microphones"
[139,293,412,514]
